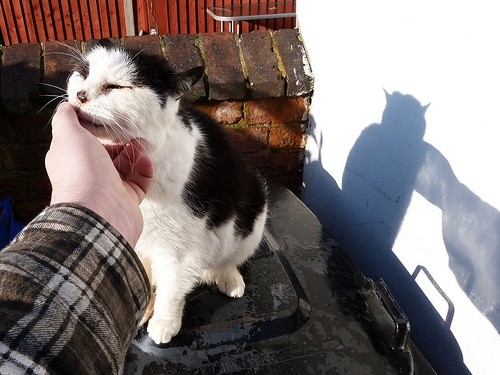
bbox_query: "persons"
[0,101,155,375]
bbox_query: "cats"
[34,32,271,345]
